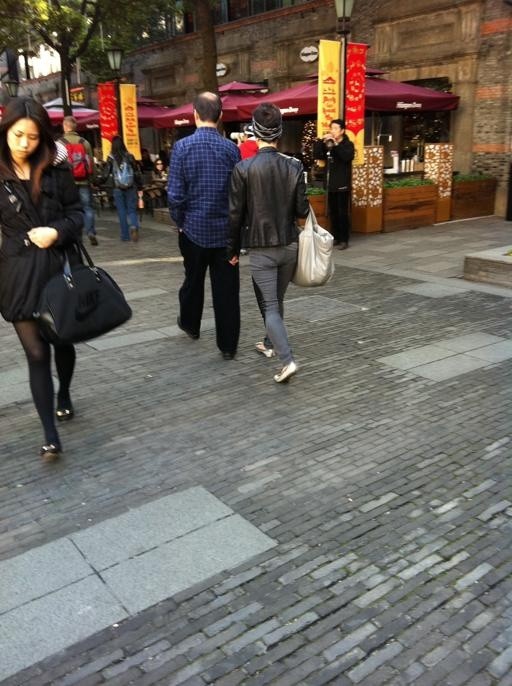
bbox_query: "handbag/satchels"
[293,202,334,288]
[34,216,133,343]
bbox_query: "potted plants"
[382,178,436,234]
[297,184,331,234]
[450,173,498,219]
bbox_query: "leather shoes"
[40,440,64,458]
[272,363,300,382]
[173,318,201,339]
[218,342,240,361]
[56,395,73,419]
[254,340,272,357]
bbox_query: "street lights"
[93,33,145,164]
[2,73,21,100]
[316,0,373,130]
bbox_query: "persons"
[310,119,356,249]
[0,96,89,458]
[54,116,178,246]
[166,91,244,360]
[226,101,310,384]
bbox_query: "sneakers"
[337,241,348,250]
[87,226,139,246]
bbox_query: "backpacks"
[104,153,138,190]
[60,137,91,179]
